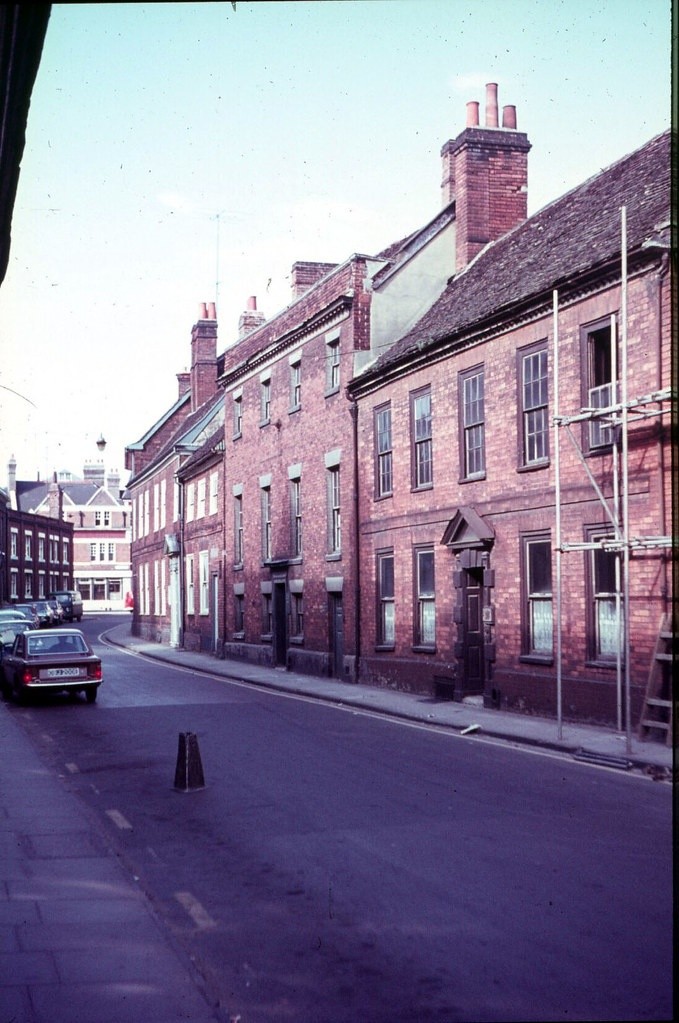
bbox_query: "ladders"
[636,611,674,747]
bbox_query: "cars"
[28,601,53,628]
[0,604,43,654]
[0,628,104,706]
[47,600,65,625]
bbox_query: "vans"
[46,590,83,624]
